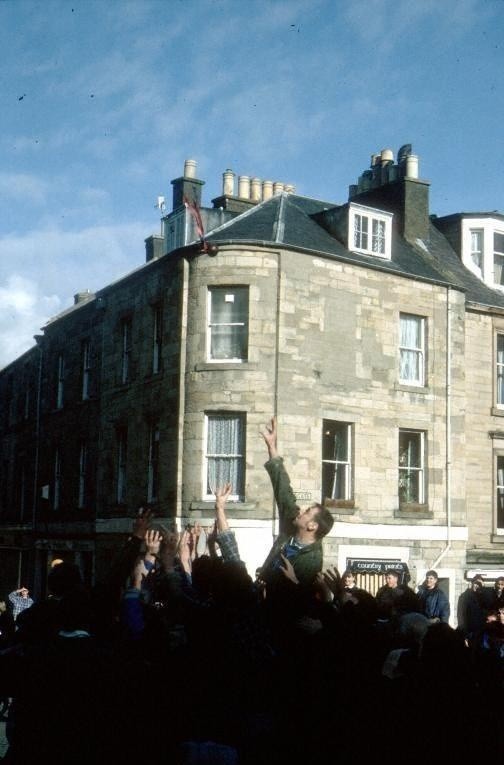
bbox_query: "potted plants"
[323,496,355,515]
[401,501,422,513]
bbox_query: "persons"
[254,415,334,584]
[213,481,243,561]
